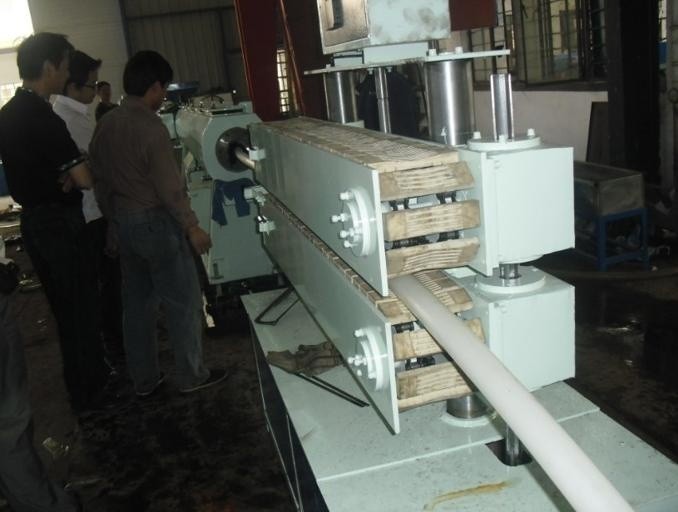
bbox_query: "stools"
[566,212,651,272]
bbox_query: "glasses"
[82,81,99,90]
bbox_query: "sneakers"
[181,368,229,392]
[136,371,165,395]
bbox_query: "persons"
[87,50,229,397]
[52,49,127,364]
[94,79,121,123]
[0,32,136,420]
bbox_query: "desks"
[240,285,678,512]
